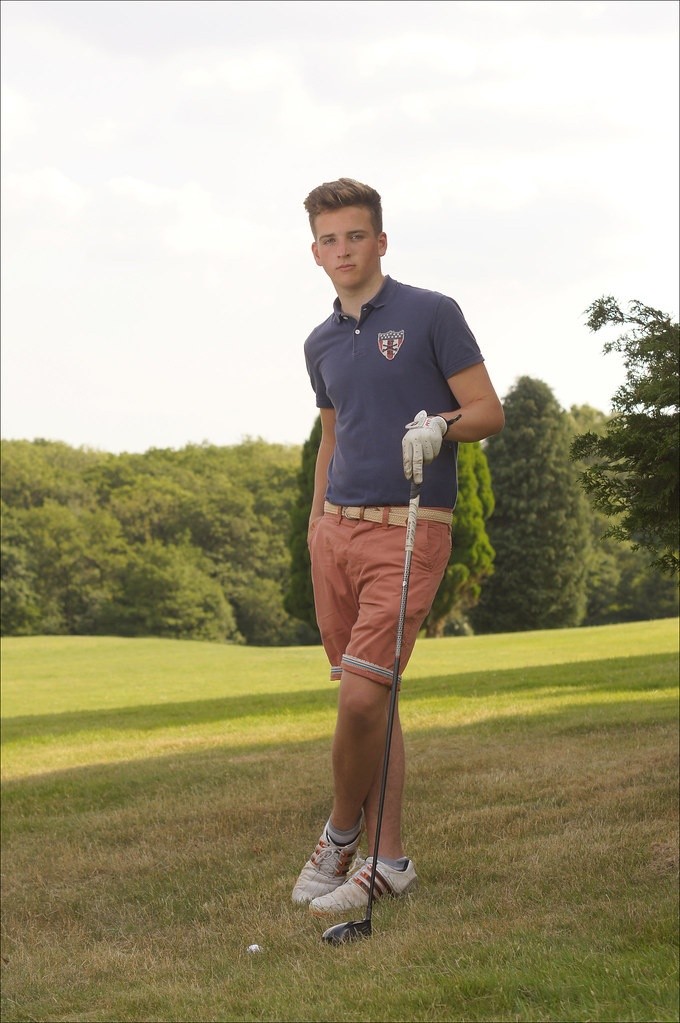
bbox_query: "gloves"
[402,410,448,484]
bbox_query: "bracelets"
[428,412,462,438]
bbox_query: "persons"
[289,178,504,914]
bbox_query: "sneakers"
[291,820,365,904]
[310,858,419,915]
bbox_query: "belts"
[324,501,453,527]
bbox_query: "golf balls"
[248,943,262,951]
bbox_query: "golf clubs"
[319,477,424,945]
